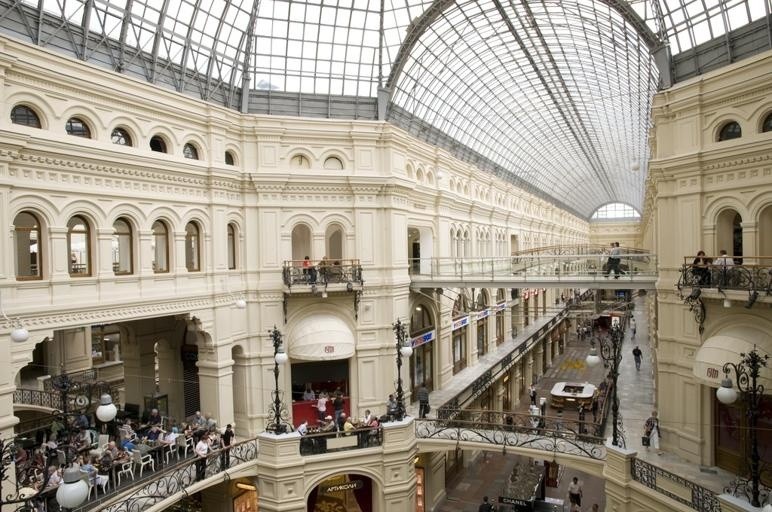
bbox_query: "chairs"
[297,417,379,453]
[685,261,740,287]
[29,416,217,512]
[293,265,345,282]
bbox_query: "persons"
[611,241,621,278]
[478,477,599,512]
[626,308,632,324]
[302,255,342,284]
[642,410,664,456]
[576,324,602,340]
[707,250,736,287]
[632,344,643,371]
[295,382,429,448]
[630,318,636,339]
[46,407,235,485]
[691,250,713,286]
[604,243,628,277]
[506,384,600,429]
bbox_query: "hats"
[324,416,332,420]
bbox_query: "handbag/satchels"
[642,431,651,446]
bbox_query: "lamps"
[230,301,246,310]
[11,317,29,343]
[630,156,652,171]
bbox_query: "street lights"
[51,362,120,512]
[265,322,295,436]
[0,429,92,512]
[386,316,415,425]
[585,321,625,450]
[714,343,772,509]
